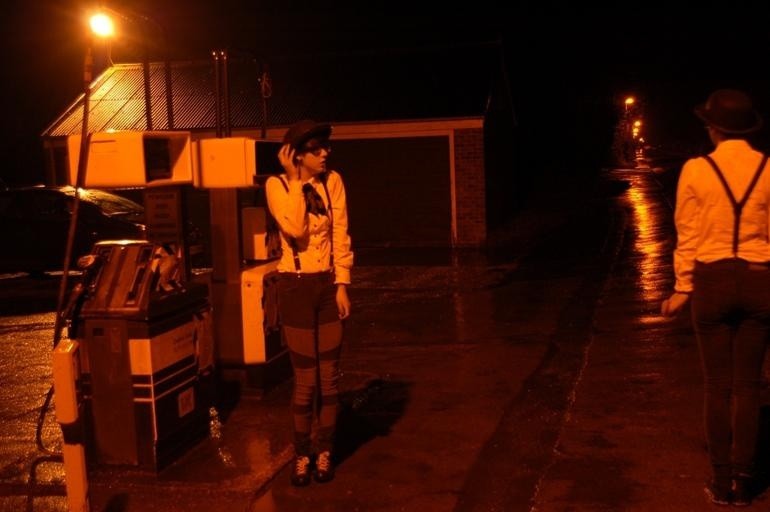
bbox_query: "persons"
[658,88,769,508]
[263,123,358,487]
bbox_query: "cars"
[1,183,205,280]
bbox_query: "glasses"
[304,138,332,155]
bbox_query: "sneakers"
[291,453,311,487]
[315,446,331,481]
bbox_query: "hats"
[282,118,332,152]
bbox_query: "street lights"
[623,94,644,156]
[89,1,174,131]
